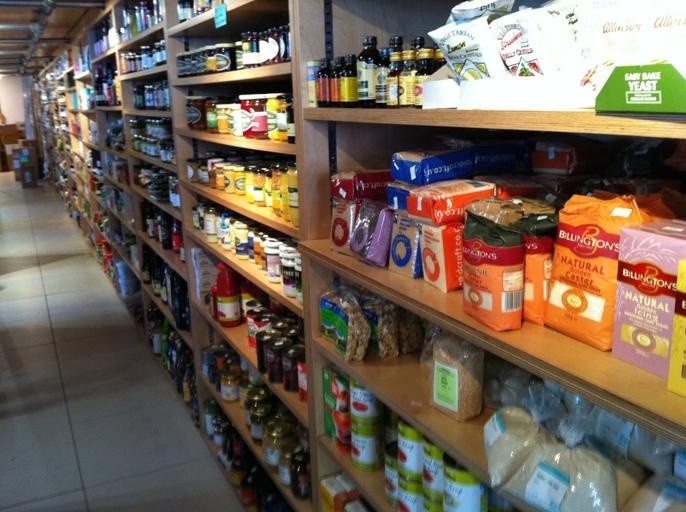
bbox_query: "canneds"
[110,0,312,500]
[320,362,489,512]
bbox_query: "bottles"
[203,387,310,512]
[243,24,292,67]
[140,173,197,427]
[212,260,259,328]
[317,35,447,109]
[117,0,174,163]
[176,0,220,27]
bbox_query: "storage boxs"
[6,139,39,188]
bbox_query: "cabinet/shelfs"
[31,0,686,512]
